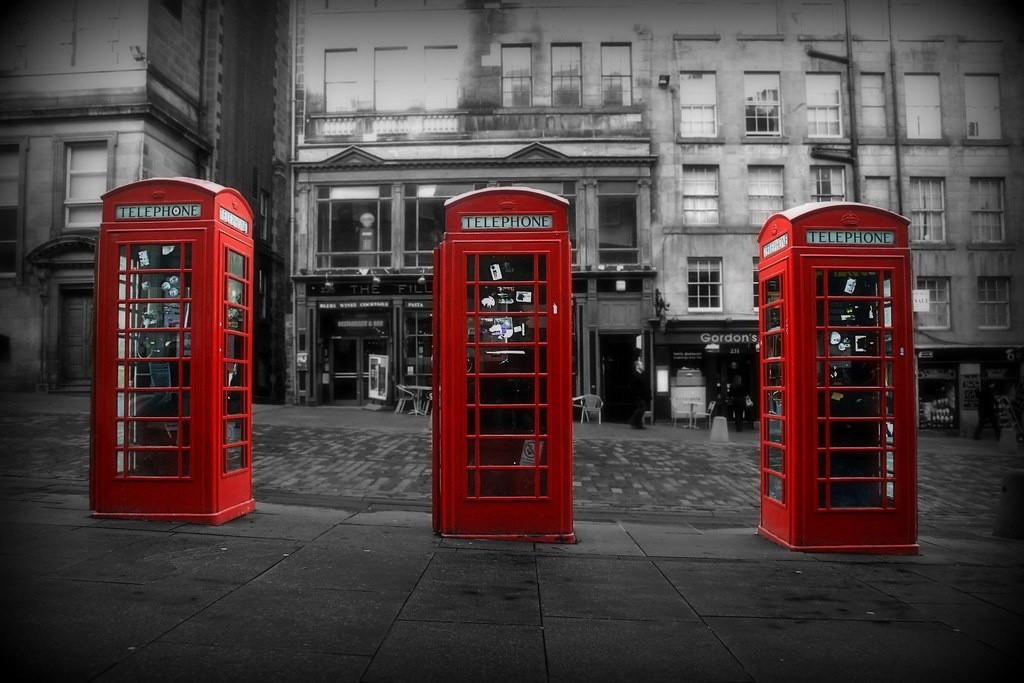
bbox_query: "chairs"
[694,400,716,431]
[670,398,690,428]
[395,385,418,417]
[581,394,603,425]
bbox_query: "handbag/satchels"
[745,395,753,407]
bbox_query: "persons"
[628,359,650,428]
[721,359,760,432]
[970,377,1003,444]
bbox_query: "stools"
[641,412,654,426]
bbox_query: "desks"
[680,401,705,430]
[402,385,432,415]
